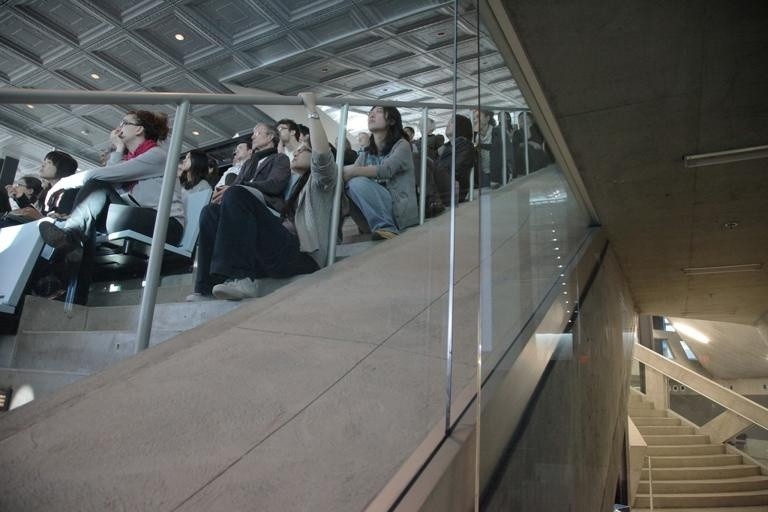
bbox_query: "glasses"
[279,128,288,131]
[119,120,137,128]
[293,148,312,156]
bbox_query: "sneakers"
[371,230,398,241]
[185,277,259,302]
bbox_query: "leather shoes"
[39,221,84,263]
[54,292,88,305]
